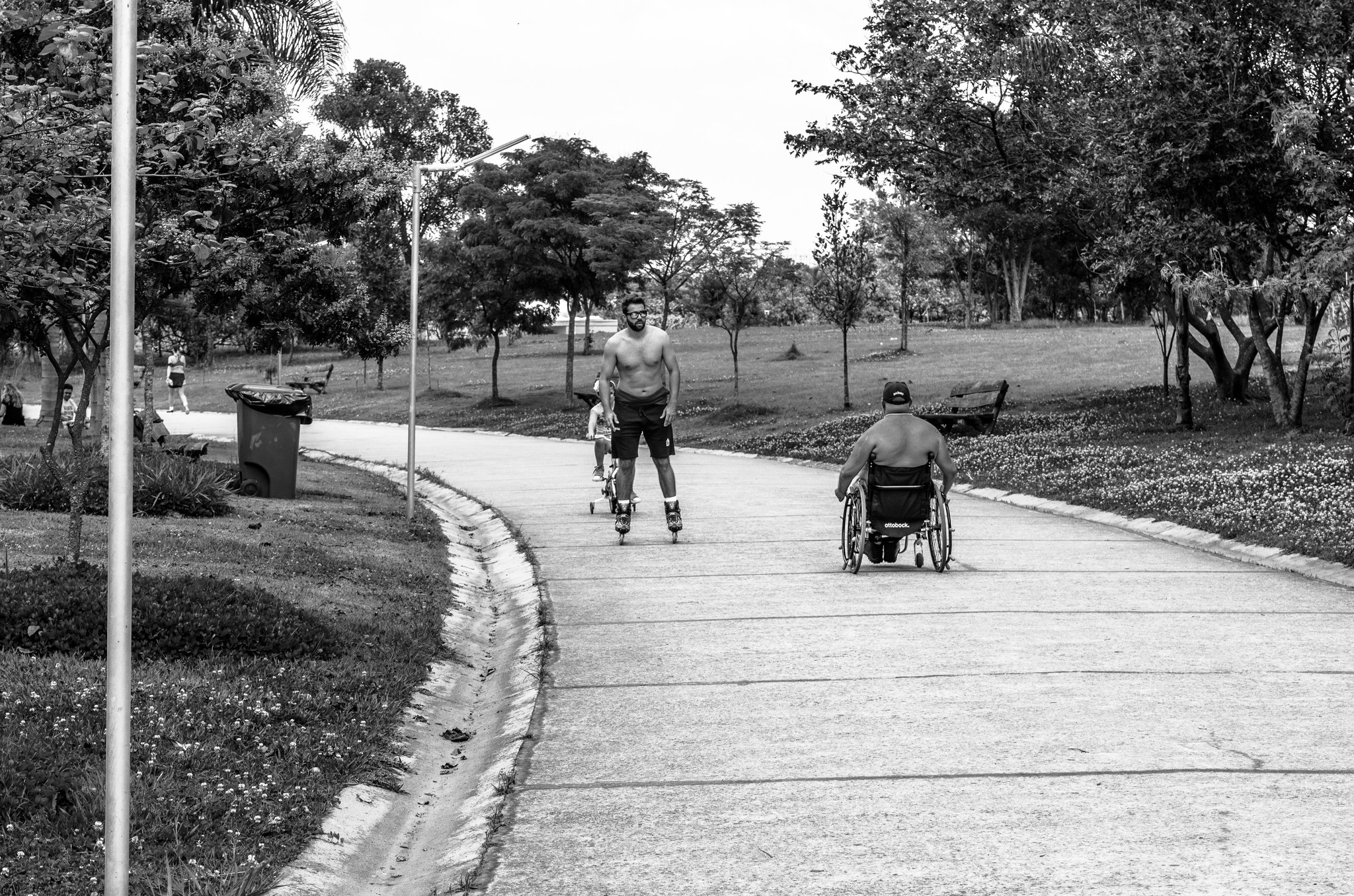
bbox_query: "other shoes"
[863,542,883,564]
[883,540,900,563]
[185,409,190,414]
[167,410,173,413]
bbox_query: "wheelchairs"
[839,451,955,574]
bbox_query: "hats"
[883,380,910,405]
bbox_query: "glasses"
[627,310,647,319]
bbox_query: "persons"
[0,383,26,427]
[586,380,642,504]
[166,345,190,414]
[599,297,683,546]
[835,380,956,564]
[61,384,89,430]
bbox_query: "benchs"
[573,372,619,408]
[136,409,209,459]
[285,363,334,394]
[915,380,1009,436]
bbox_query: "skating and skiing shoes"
[615,500,632,545]
[664,499,683,544]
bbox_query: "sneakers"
[592,465,604,481]
[630,490,641,504]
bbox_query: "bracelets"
[166,376,169,379]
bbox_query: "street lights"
[404,134,534,518]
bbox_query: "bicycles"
[586,433,636,515]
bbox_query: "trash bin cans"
[227,385,313,500]
